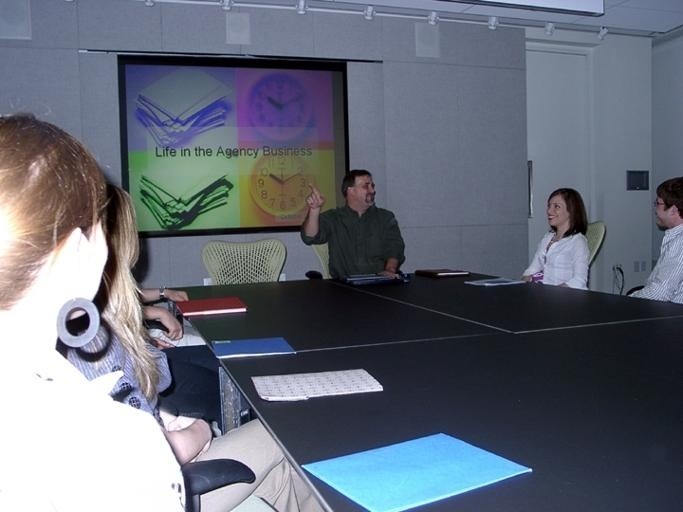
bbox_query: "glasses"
[654,201,667,208]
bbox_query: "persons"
[626,175,682,304]
[519,187,590,292]
[299,169,406,280]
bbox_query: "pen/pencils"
[537,282,544,288]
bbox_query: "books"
[248,367,383,402]
[343,272,386,280]
[301,432,532,512]
[415,267,470,278]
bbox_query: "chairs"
[184,459,256,511]
[202,238,287,285]
[314,243,333,279]
[586,220,606,264]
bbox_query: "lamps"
[145,0,608,38]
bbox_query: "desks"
[167,271,683,512]
